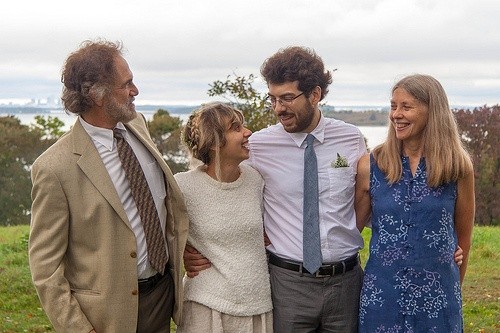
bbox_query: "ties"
[113,131,168,276]
[302,135,323,274]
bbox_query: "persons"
[28,41,190,333]
[265,73,476,333]
[174,102,274,332]
[183,46,464,333]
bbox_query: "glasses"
[265,92,306,107]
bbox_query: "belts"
[268,254,358,278]
[138,260,173,290]
